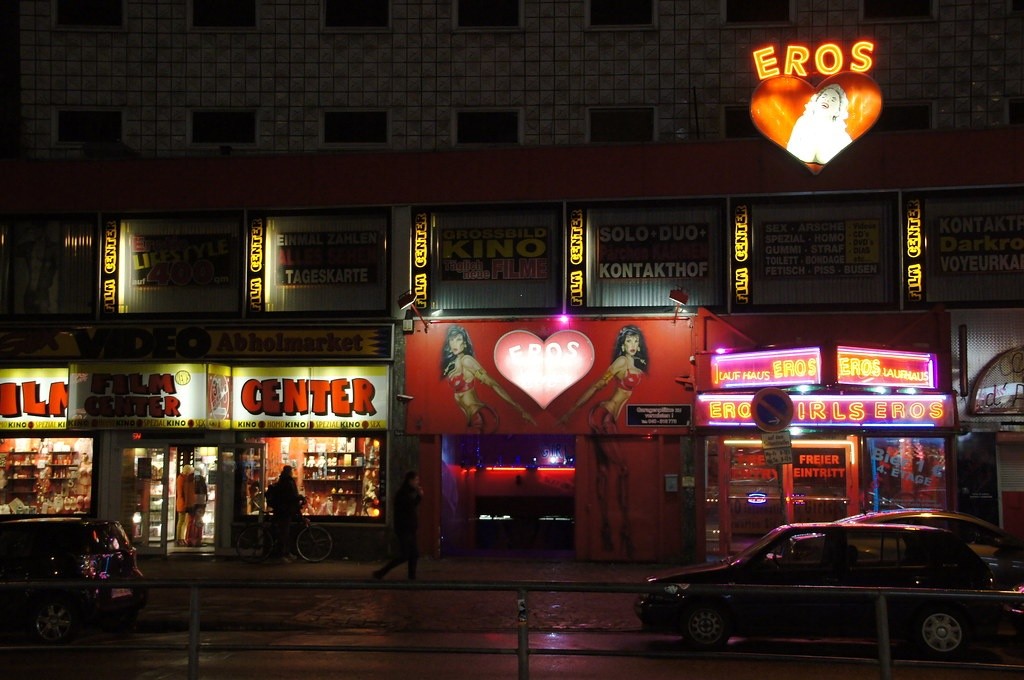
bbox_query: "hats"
[183,465,194,475]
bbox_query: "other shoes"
[285,552,298,560]
[195,544,206,547]
[272,557,292,564]
[176,540,186,545]
[187,543,192,547]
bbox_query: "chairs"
[847,544,859,580]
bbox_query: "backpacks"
[266,483,284,508]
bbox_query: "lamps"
[398,290,430,333]
[669,285,689,323]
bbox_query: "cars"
[633,521,1005,661]
[781,507,1024,596]
[0,512,143,646]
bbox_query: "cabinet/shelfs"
[302,451,364,498]
[8,451,78,500]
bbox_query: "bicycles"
[235,501,334,564]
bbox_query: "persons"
[373,468,425,581]
[176,464,207,547]
[273,465,307,565]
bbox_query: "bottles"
[259,508,263,523]
